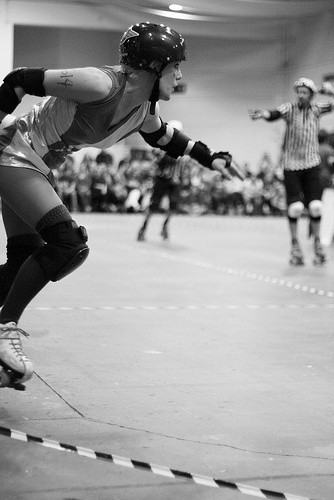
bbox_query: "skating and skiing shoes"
[0,305,34,391]
[314,247,325,262]
[290,248,303,264]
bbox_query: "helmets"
[119,22,186,70]
[166,120,183,132]
[293,78,316,93]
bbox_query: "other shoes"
[161,230,168,240]
[138,228,144,239]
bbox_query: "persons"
[0,22,246,391]
[248,77,334,266]
[137,119,191,242]
[52,144,287,217]
[312,73,334,248]
